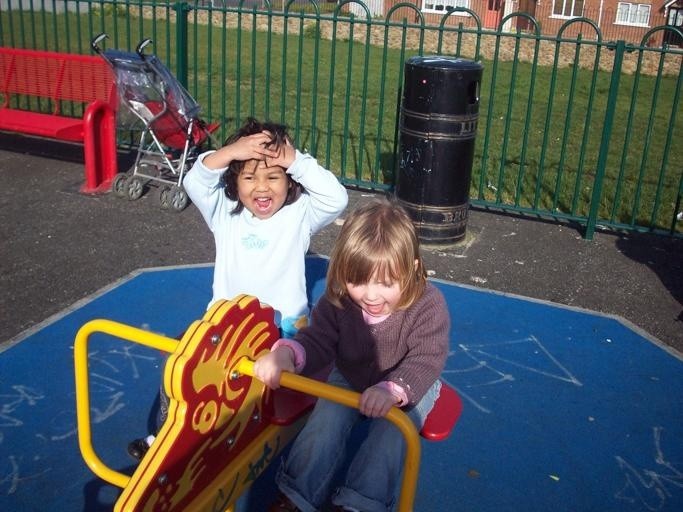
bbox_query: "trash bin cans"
[392,56,483,246]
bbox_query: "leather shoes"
[126,430,158,461]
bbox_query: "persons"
[126,117,348,460]
[252,206,450,512]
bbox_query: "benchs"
[0,47,121,192]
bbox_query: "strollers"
[91,33,224,213]
